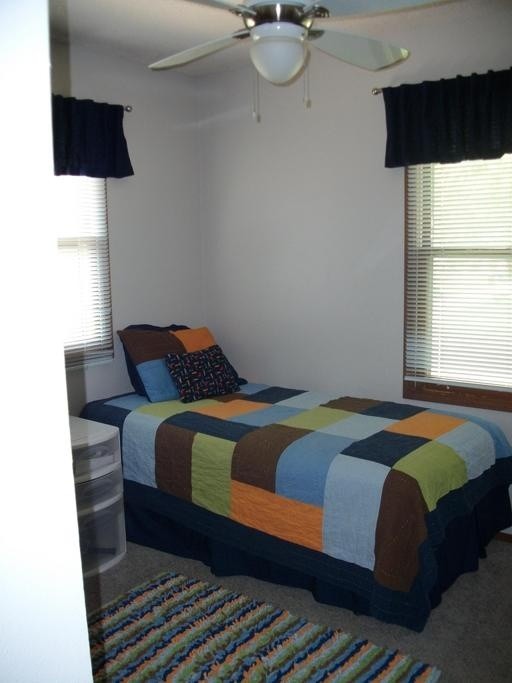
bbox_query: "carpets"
[83,568,447,682]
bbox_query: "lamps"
[247,2,311,86]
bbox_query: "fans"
[145,2,412,75]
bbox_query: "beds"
[78,371,512,634]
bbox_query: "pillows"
[115,318,194,398]
[115,323,218,404]
[163,346,239,403]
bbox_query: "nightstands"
[69,409,129,580]
